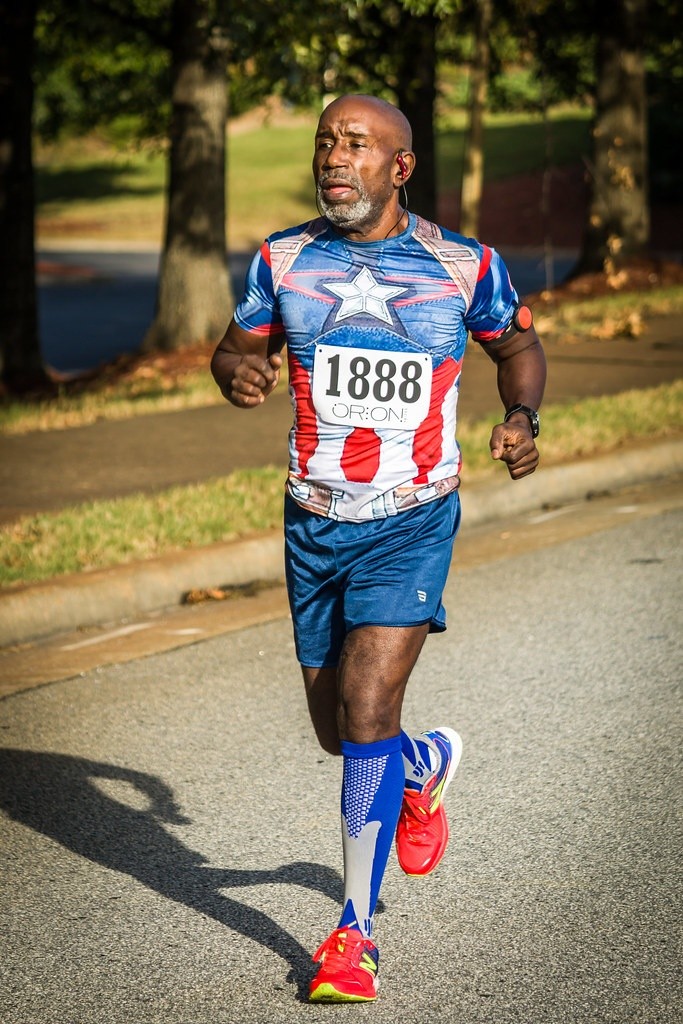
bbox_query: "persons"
[210,97,545,1004]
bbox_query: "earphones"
[396,155,408,179]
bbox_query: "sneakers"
[394,727,463,877]
[311,928,381,1004]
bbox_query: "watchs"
[505,404,540,438]
